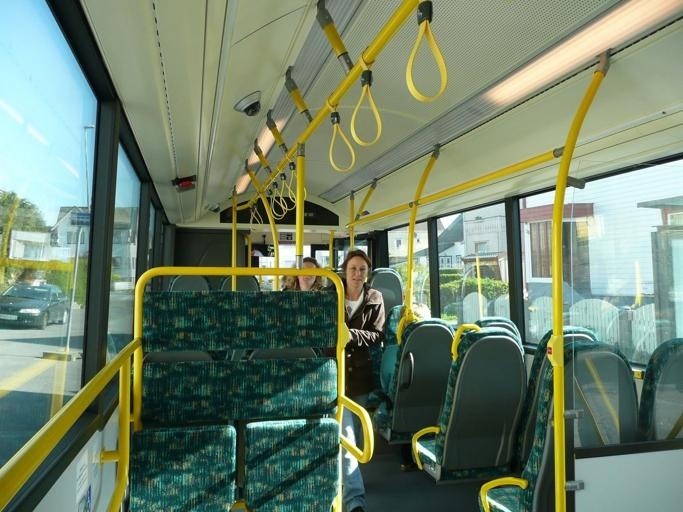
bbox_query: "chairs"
[102,265,683,511]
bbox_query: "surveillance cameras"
[234,91,262,118]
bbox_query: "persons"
[337,249,387,511]
[281,256,332,293]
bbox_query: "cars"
[0,280,70,330]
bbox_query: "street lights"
[82,124,94,214]
